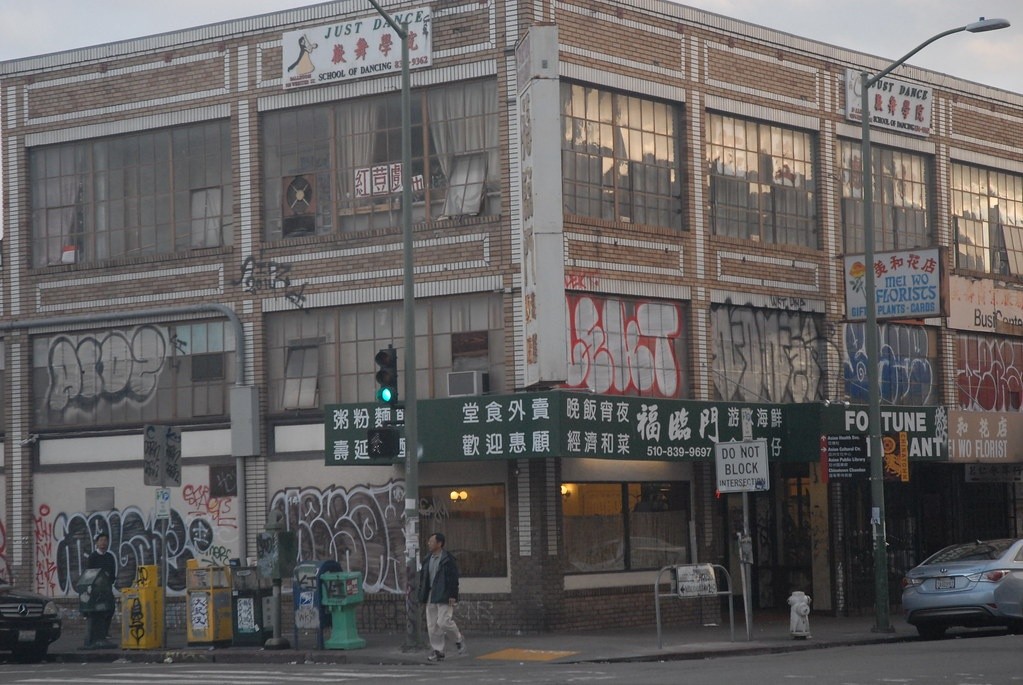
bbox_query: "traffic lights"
[369,428,400,460]
[375,344,398,406]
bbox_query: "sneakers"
[453,634,466,656]
[428,649,446,664]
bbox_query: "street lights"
[854,14,1010,631]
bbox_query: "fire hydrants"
[785,591,814,639]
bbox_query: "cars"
[901,536,1023,639]
[0,577,62,666]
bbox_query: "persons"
[85,531,119,645]
[413,532,467,662]
